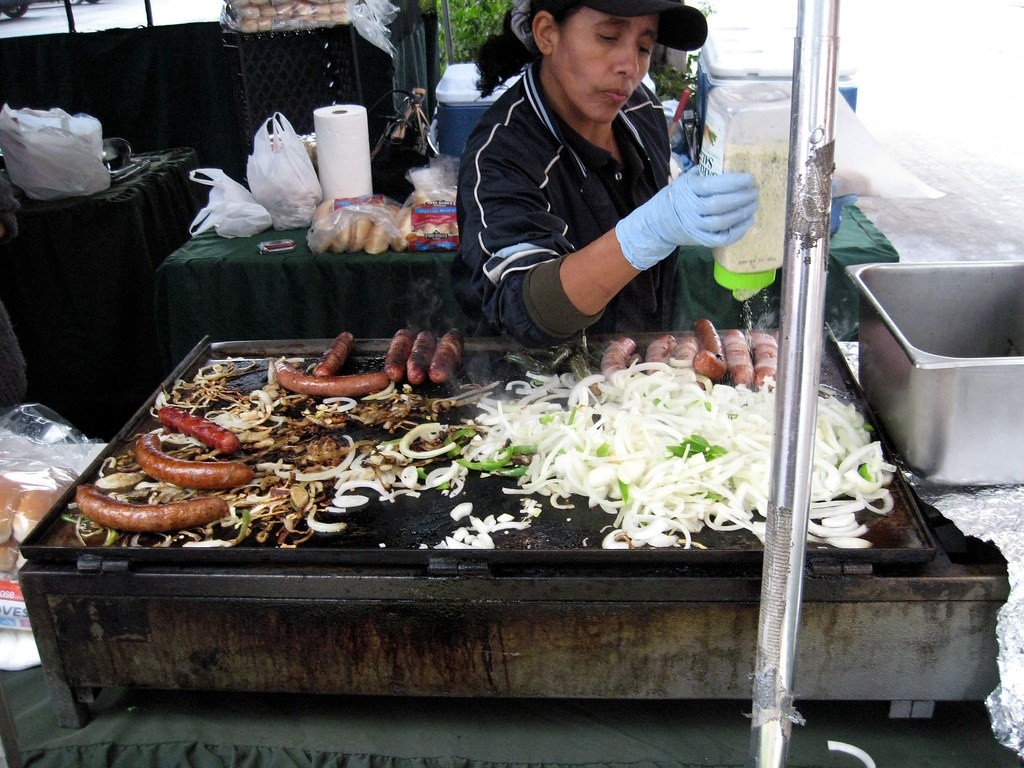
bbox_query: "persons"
[449,0,759,387]
[0,175,27,410]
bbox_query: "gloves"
[615,160,759,271]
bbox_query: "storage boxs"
[845,257,1024,486]
[694,25,856,230]
[435,62,526,159]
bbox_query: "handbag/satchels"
[246,111,323,231]
[189,168,273,238]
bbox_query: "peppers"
[502,326,604,384]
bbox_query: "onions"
[208,357,898,552]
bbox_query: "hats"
[530,0,707,51]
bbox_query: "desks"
[1,148,204,394]
[153,225,746,334]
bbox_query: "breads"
[309,189,459,254]
[231,0,349,34]
[0,461,79,572]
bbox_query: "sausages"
[76,484,229,533]
[273,328,465,397]
[136,433,254,489]
[158,406,240,454]
[602,319,782,390]
